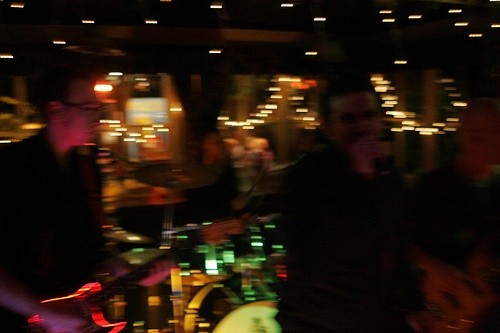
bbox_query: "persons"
[177,126,248,240]
[280,74,417,333]
[413,98,499,333]
[1,50,174,333]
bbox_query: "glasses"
[59,99,107,113]
[338,109,377,125]
